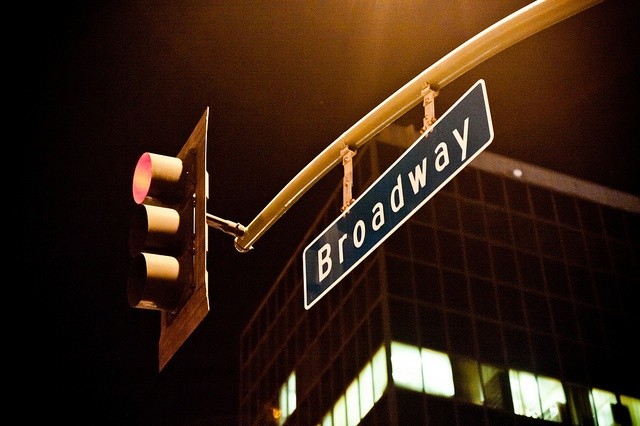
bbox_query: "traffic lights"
[124,106,210,375]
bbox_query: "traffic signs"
[303,78,494,310]
